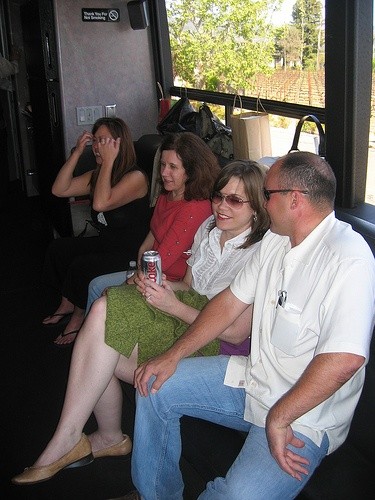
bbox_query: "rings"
[142,290,145,296]
[147,296,152,300]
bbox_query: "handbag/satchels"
[157,96,273,161]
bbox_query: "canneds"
[141,250,162,286]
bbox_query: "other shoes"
[110,489,143,500]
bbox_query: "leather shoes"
[87,433,133,458]
[11,433,94,486]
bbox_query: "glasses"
[262,186,309,202]
[210,191,258,210]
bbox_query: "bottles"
[126,261,138,282]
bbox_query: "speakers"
[127,0,150,31]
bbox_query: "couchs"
[136,133,375,500]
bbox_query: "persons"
[85,132,222,313]
[10,160,270,485]
[0,48,22,91]
[41,116,153,347]
[109,151,375,500]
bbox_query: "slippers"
[42,311,67,326]
[55,329,79,346]
